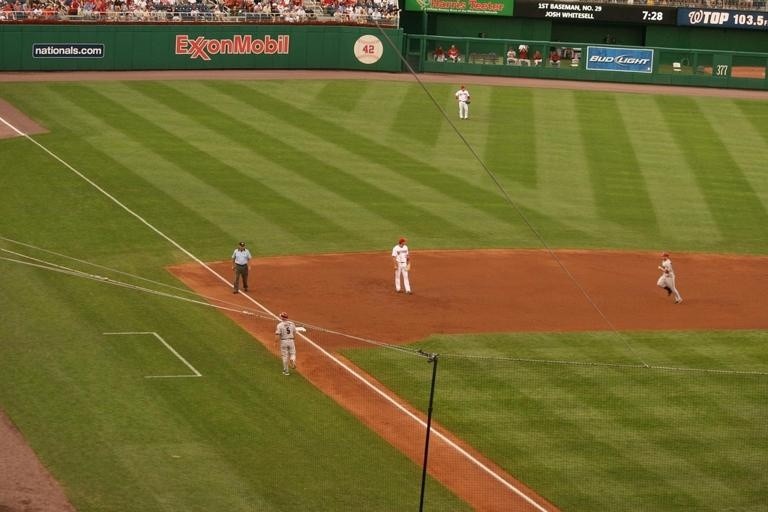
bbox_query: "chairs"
[672,62,681,73]
[426,52,503,65]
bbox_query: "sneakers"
[244,287,247,291]
[233,291,239,294]
[281,371,290,376]
[674,299,683,304]
[667,289,673,296]
[406,290,412,294]
[396,289,404,293]
[288,358,296,368]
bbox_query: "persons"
[1,1,399,28]
[391,238,413,295]
[433,42,572,69]
[273,311,297,376]
[656,251,683,305]
[454,85,471,120]
[230,242,252,294]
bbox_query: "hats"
[279,311,289,320]
[659,251,669,257]
[239,242,246,246]
[399,238,408,244]
